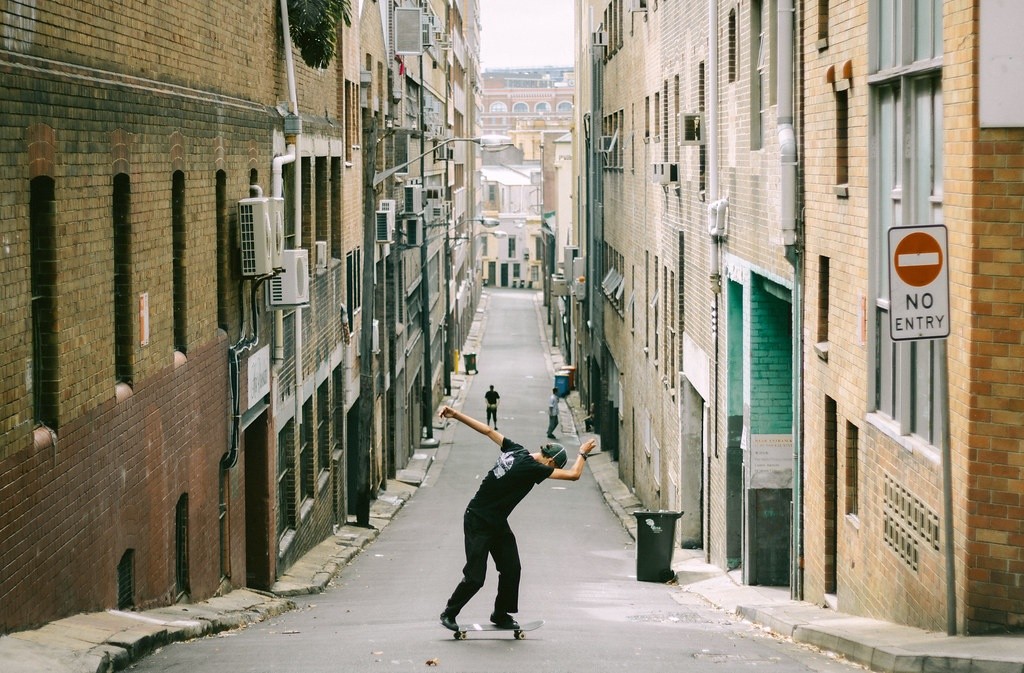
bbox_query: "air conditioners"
[237,197,327,312]
[424,94,440,114]
[593,31,609,47]
[652,162,680,184]
[597,136,614,152]
[422,1,452,52]
[375,184,453,248]
[678,110,708,145]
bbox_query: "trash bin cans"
[553,365,576,397]
[627,509,685,583]
[463,352,478,375]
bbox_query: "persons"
[547,387,560,439]
[438,407,597,630]
[485,385,500,430]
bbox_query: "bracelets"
[578,452,588,461]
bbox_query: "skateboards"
[438,619,546,640]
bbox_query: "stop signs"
[894,232,944,288]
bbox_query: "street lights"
[356,135,515,528]
[420,217,501,439]
[445,230,506,397]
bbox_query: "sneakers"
[440,612,459,631]
[490,613,520,630]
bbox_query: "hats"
[542,443,567,469]
